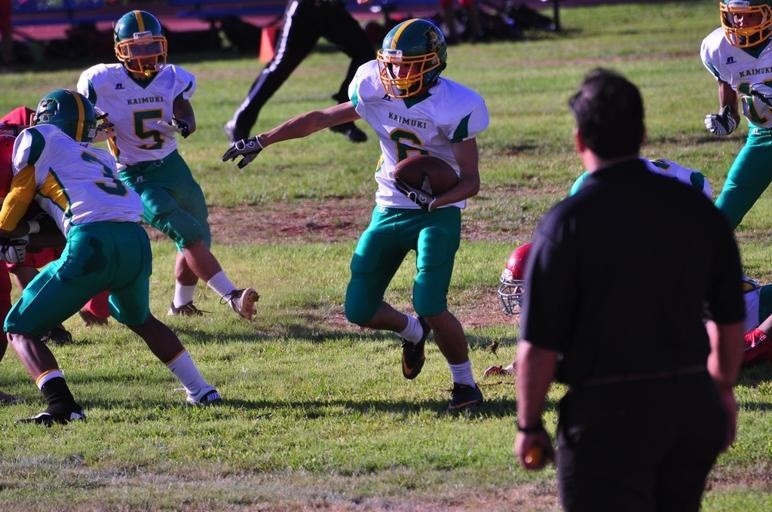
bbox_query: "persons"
[692,0,771,232]
[483,240,772,378]
[0,87,223,427]
[220,17,490,412]
[511,71,748,511]
[0,104,117,409]
[76,10,259,321]
[224,0,377,144]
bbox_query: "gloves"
[221,136,264,169]
[156,119,190,139]
[392,177,436,214]
[705,107,737,135]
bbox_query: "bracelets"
[514,419,543,434]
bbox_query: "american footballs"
[393,155,460,197]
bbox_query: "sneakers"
[220,288,259,318]
[41,325,72,346]
[402,317,431,379]
[331,122,370,145]
[186,388,222,406]
[448,383,483,411]
[19,407,86,426]
[221,122,251,145]
[168,301,204,317]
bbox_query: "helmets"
[33,90,96,148]
[720,0,772,47]
[113,12,168,76]
[376,21,448,98]
[500,244,532,318]
